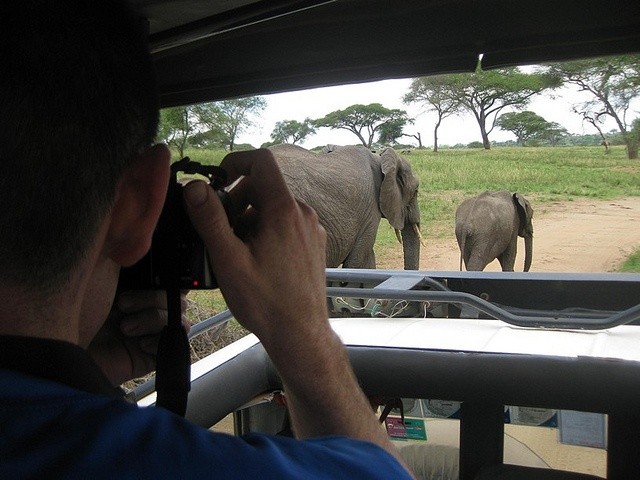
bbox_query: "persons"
[1,3,419,480]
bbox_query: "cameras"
[117,157,229,290]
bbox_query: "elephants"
[455,190,535,272]
[265,142,427,270]
